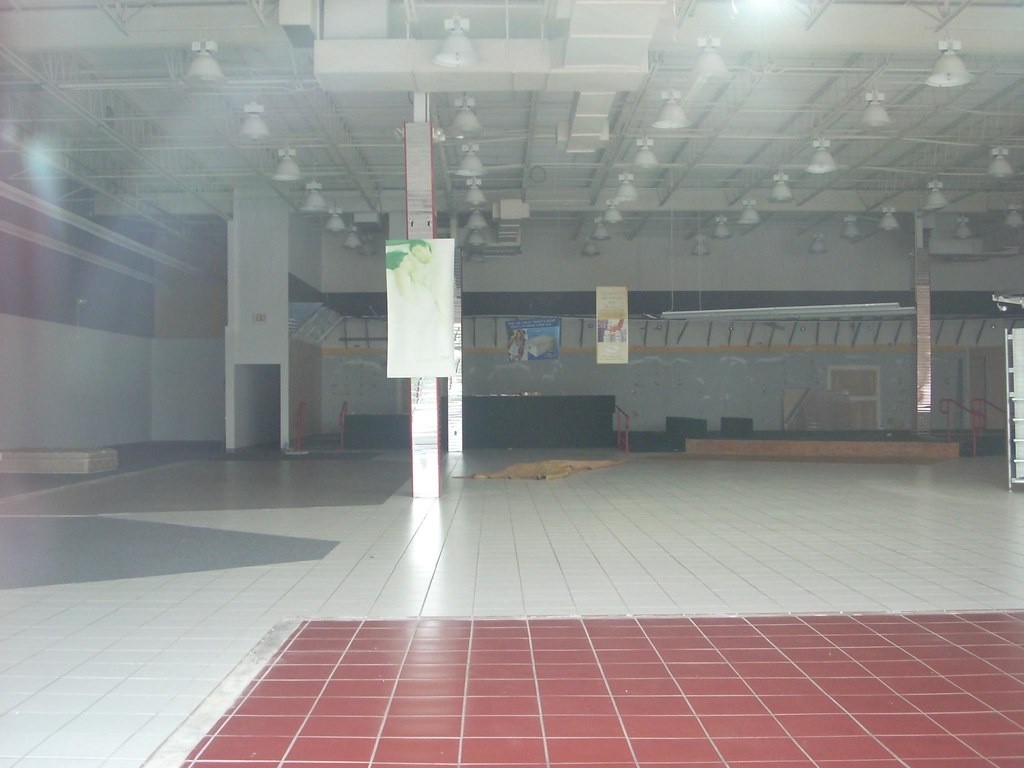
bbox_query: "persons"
[507,330,528,362]
[386,240,455,331]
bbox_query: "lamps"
[861,84,893,126]
[925,40,972,86]
[452,144,490,246]
[447,91,484,140]
[877,206,900,231]
[988,145,1012,178]
[952,213,973,240]
[432,9,478,68]
[271,148,378,256]
[184,35,227,83]
[806,213,861,255]
[690,199,760,256]
[691,35,727,78]
[236,101,272,140]
[922,176,950,209]
[650,87,691,129]
[767,168,794,203]
[1002,202,1024,228]
[582,136,658,258]
[806,137,838,174]
